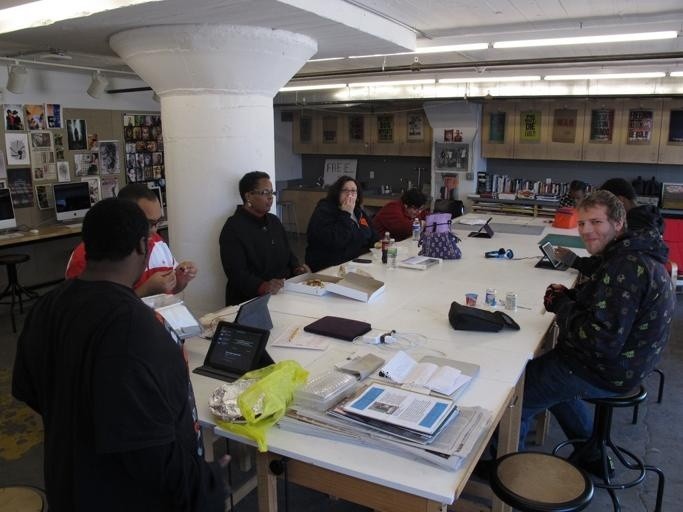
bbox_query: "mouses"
[30,229,39,233]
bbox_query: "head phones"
[485,248,513,259]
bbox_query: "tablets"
[234,292,270,321]
[204,321,270,375]
[540,241,562,268]
[478,217,492,233]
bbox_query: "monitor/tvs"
[53,182,91,224]
[0,188,17,230]
[149,187,164,217]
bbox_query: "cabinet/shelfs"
[481,98,683,165]
[292,109,431,157]
[279,187,430,226]
[467,194,560,218]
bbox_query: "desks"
[0,224,82,300]
[184,212,593,511]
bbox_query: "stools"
[0,485,49,511]
[489,451,595,512]
[1,254,40,333]
[552,385,665,512]
[276,201,300,238]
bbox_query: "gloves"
[552,245,577,266]
[543,284,569,312]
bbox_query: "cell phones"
[353,259,371,263]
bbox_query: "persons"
[515,192,674,479]
[603,177,666,260]
[305,177,378,273]
[10,197,233,512]
[219,170,305,307]
[557,177,588,214]
[369,190,431,242]
[63,182,196,300]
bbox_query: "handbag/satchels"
[418,231,462,259]
[425,213,452,231]
[449,300,519,332]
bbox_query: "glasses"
[251,189,279,196]
[340,189,357,193]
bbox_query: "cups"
[504,293,517,310]
[463,292,478,308]
[511,179,568,197]
[484,289,496,307]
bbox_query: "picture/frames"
[81,176,102,206]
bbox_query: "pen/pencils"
[288,327,300,342]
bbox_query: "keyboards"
[0,232,24,240]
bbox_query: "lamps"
[7,60,29,94]
[152,92,161,102]
[87,71,108,99]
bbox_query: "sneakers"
[582,455,614,479]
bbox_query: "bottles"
[387,239,396,270]
[412,218,420,241]
[381,231,390,263]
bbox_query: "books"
[286,307,484,463]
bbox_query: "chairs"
[433,199,464,219]
[663,219,683,291]
